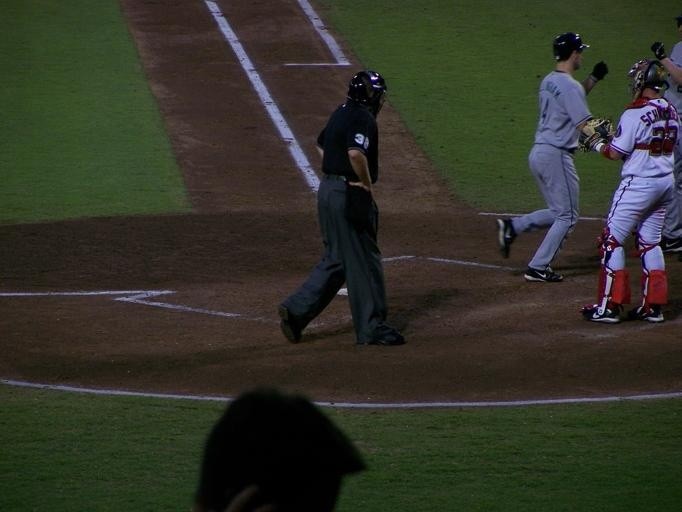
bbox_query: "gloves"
[651,42,667,60]
[589,61,608,83]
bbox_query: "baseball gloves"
[579,119,613,152]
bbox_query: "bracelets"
[589,76,597,83]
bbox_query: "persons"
[496,33,614,283]
[190,386,365,510]
[277,70,409,345]
[651,14,682,256]
[577,56,678,324]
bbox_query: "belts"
[568,148,574,153]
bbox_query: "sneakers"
[278,303,302,344]
[659,237,682,253]
[582,305,622,323]
[495,218,517,259]
[357,325,404,345]
[632,305,665,322]
[524,263,564,282]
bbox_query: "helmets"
[346,71,387,119]
[675,13,682,20]
[553,32,587,60]
[627,58,670,104]
